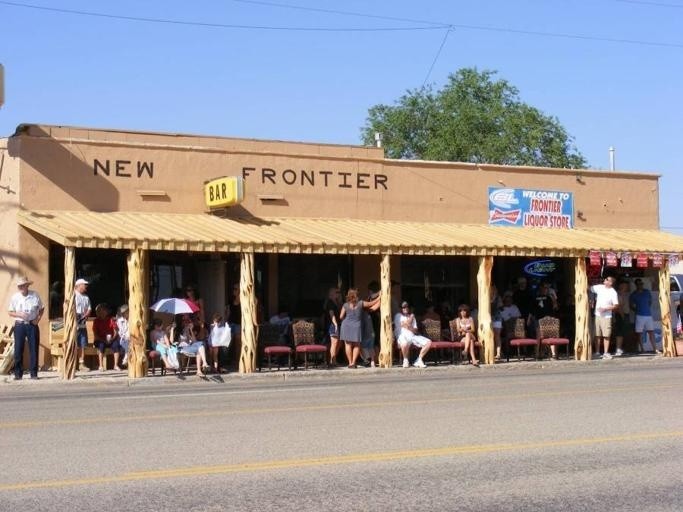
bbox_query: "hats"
[76,279,89,285]
[17,277,31,286]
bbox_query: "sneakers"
[403,359,426,368]
[602,348,623,359]
[197,366,210,377]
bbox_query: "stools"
[396,345,417,364]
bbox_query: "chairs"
[538,315,569,361]
[420,312,452,364]
[258,317,293,372]
[173,327,207,376]
[292,320,327,371]
[145,328,177,377]
[449,319,480,363]
[506,319,539,362]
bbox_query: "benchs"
[50,320,121,372]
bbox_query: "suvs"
[668,274,683,337]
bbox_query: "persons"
[91,273,682,379]
[70,276,93,372]
[7,274,45,380]
